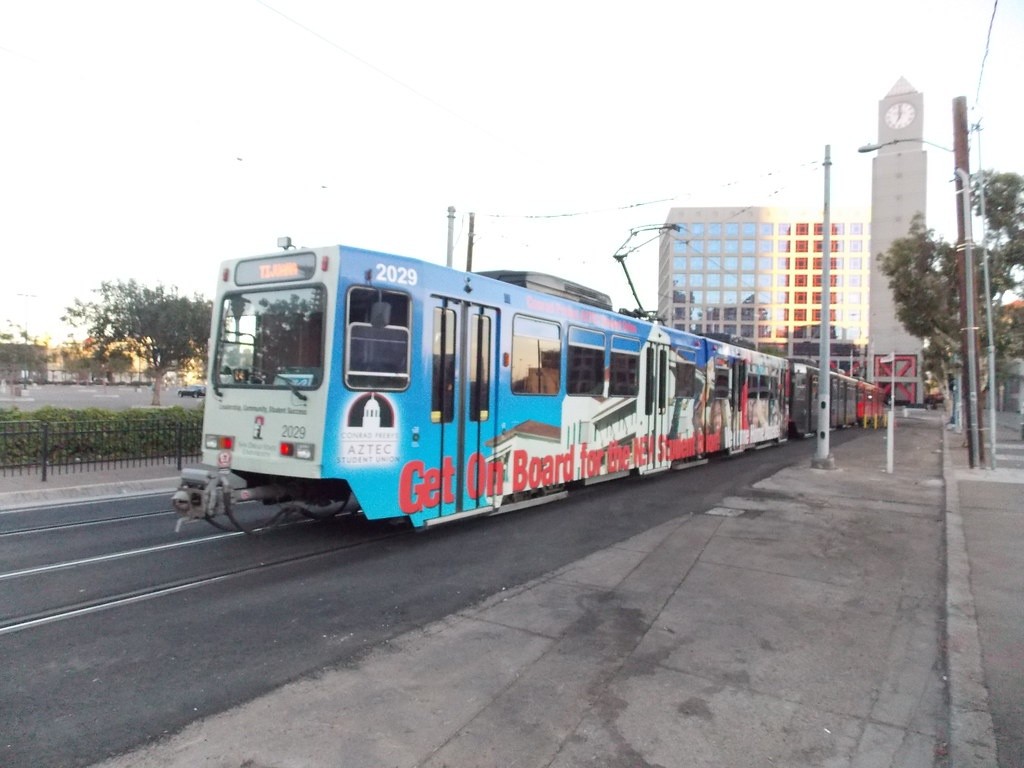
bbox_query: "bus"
[170,223,886,532]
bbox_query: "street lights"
[856,96,985,468]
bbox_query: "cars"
[177,386,206,398]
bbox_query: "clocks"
[885,101,916,129]
